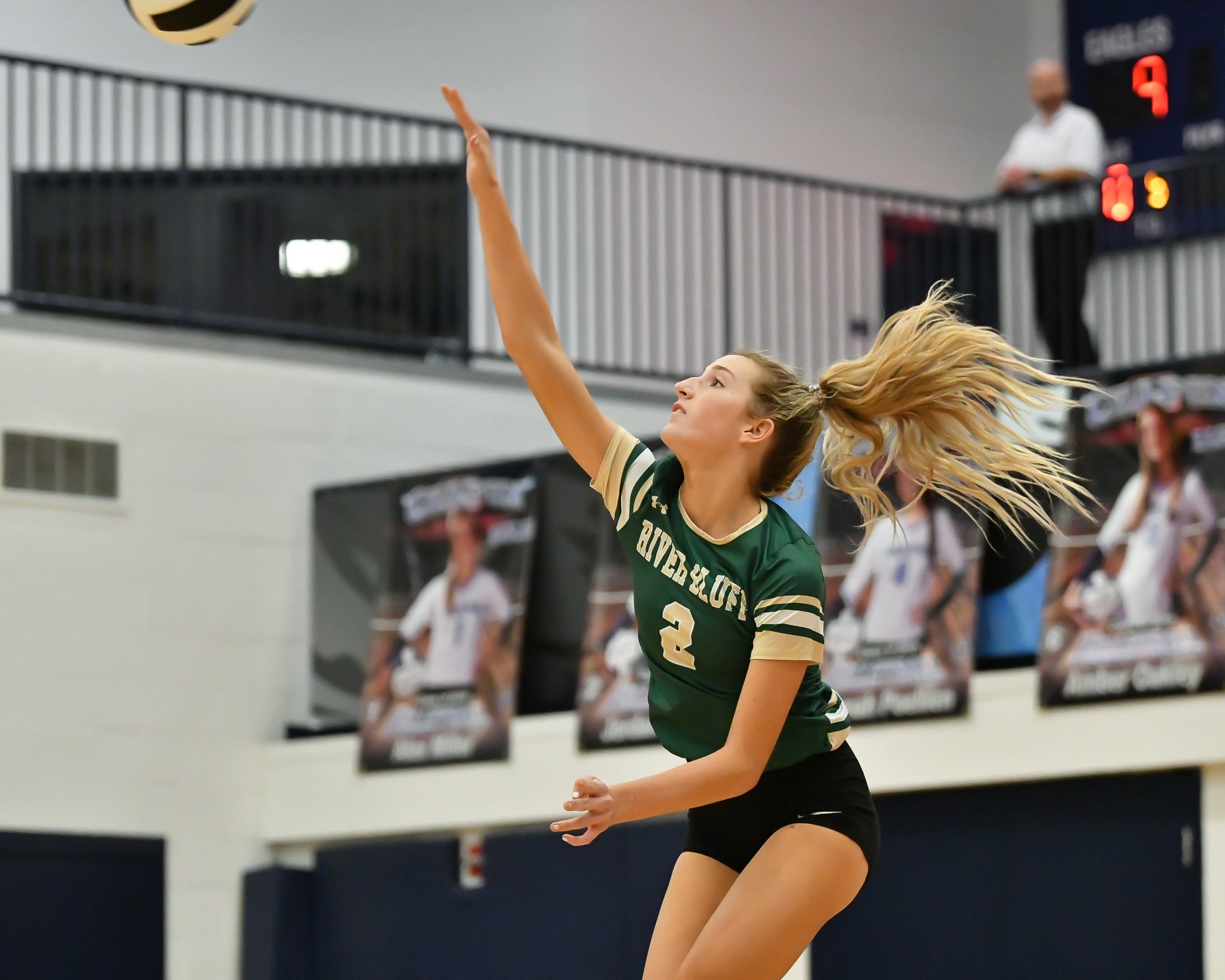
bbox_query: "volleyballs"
[123,0,259,46]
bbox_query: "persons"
[361,402,1225,758]
[999,60,1108,378]
[440,84,1127,980]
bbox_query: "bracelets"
[1031,170,1041,186]
